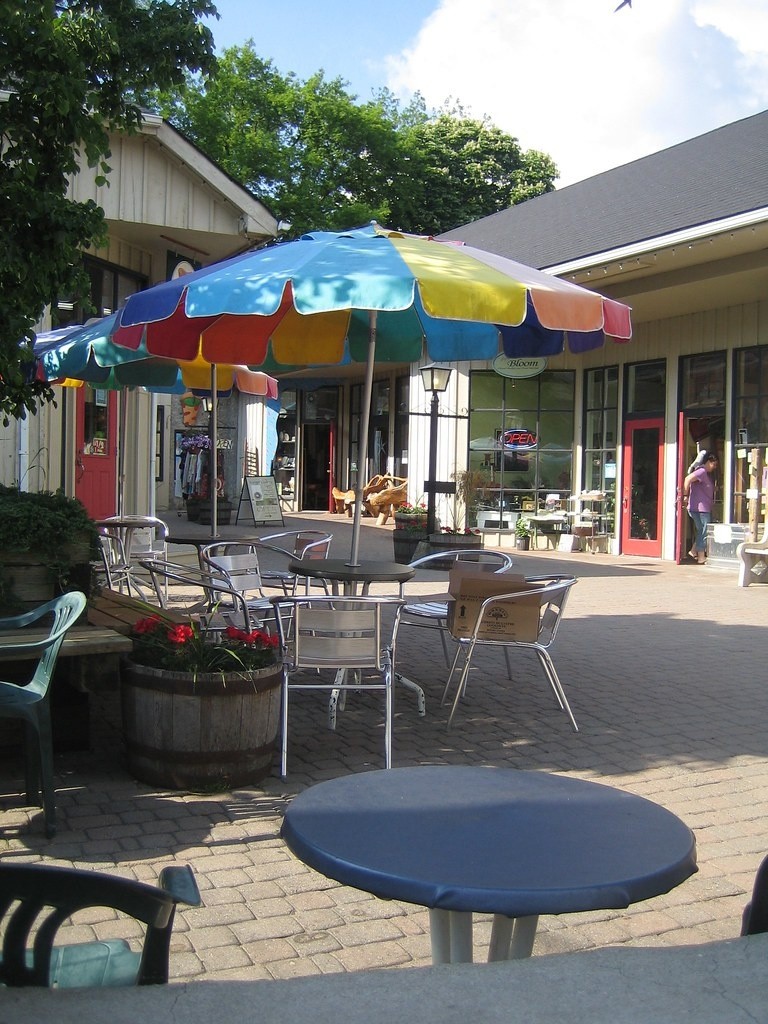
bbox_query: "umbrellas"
[20,302,280,608]
[106,221,634,731]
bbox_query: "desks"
[163,532,262,615]
[93,518,161,598]
[280,763,700,967]
[287,557,416,695]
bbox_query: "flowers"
[178,429,212,451]
[440,525,480,536]
[109,595,282,670]
[395,520,427,532]
[397,501,428,514]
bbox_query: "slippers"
[697,560,706,565]
[686,551,698,562]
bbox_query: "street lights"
[419,362,452,535]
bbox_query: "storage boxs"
[446,565,545,645]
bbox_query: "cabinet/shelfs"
[272,413,297,501]
[566,492,608,554]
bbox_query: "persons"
[682,452,719,565]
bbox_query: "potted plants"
[514,518,532,551]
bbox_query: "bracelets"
[683,494,688,497]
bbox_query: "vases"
[118,651,285,794]
[188,445,201,455]
[393,529,427,565]
[427,532,482,572]
[394,513,428,530]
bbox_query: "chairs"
[384,548,513,683]
[269,594,406,779]
[438,572,580,735]
[199,539,339,676]
[91,531,147,604]
[240,530,336,611]
[0,591,87,840]
[104,514,172,603]
[0,861,202,989]
[137,556,253,638]
